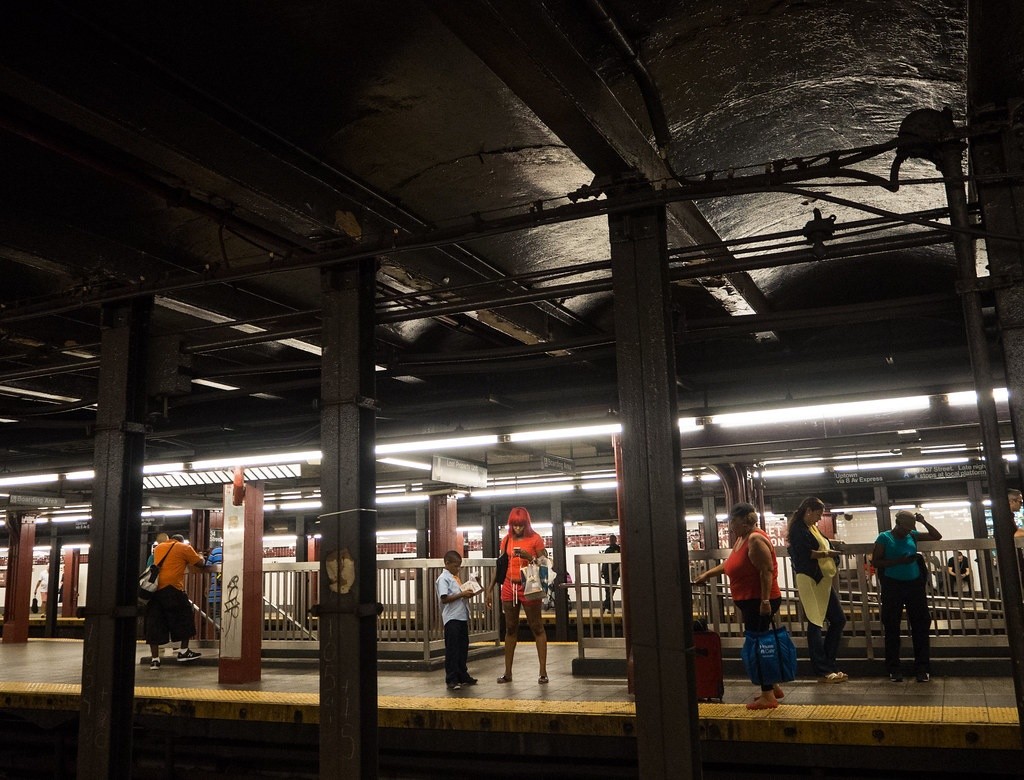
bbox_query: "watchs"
[762,599,770,605]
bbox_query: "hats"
[894,511,917,531]
[722,502,756,521]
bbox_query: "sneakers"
[177,648,202,662]
[150,658,161,670]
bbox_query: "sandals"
[538,674,549,685]
[497,674,512,683]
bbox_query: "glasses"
[1011,498,1023,505]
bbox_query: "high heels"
[818,671,849,684]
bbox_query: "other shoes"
[888,668,904,683]
[746,688,785,709]
[914,670,930,683]
[40,613,46,618]
[459,677,479,687]
[446,681,461,691]
[601,603,605,613]
[607,609,615,614]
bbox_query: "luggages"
[689,582,725,704]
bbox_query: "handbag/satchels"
[739,611,799,687]
[31,598,39,614]
[138,564,160,601]
[519,558,549,602]
[495,535,509,586]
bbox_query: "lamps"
[0,385,1011,486]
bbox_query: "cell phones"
[513,547,521,555]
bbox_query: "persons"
[864,554,881,592]
[549,565,572,587]
[871,511,942,682]
[486,508,547,684]
[145,532,223,670]
[601,535,621,614]
[34,563,66,618]
[694,500,784,709]
[1007,487,1024,595]
[786,496,855,682]
[434,550,477,690]
[947,552,972,596]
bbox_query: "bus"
[0,439,1024,613]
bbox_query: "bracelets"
[530,558,535,564]
[823,551,825,558]
[965,573,966,576]
[836,541,841,548]
